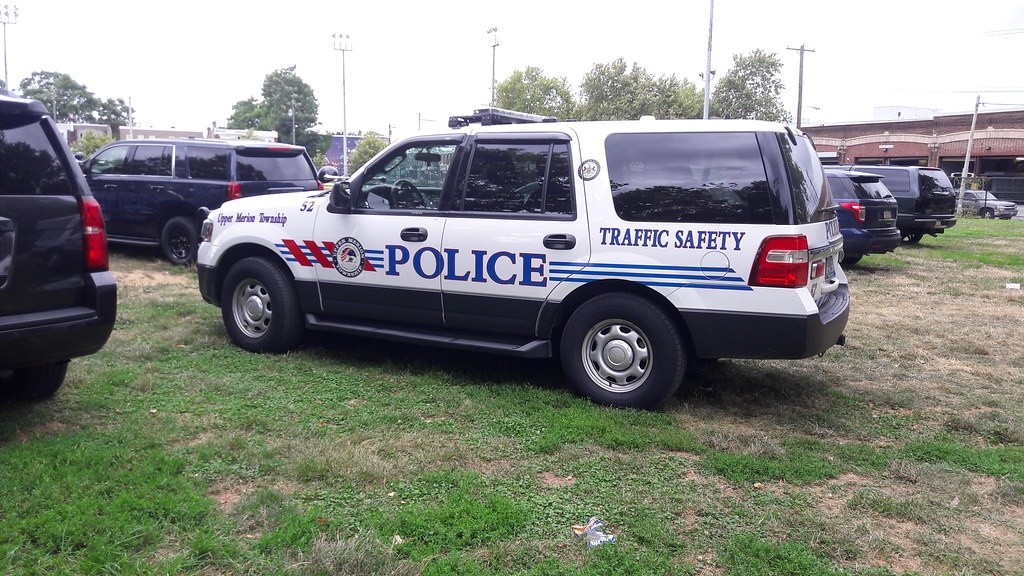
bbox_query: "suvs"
[822,168,903,265]
[76,137,326,267]
[0,88,118,406]
[955,189,1018,221]
[821,165,957,245]
[950,172,982,189]
[197,109,853,413]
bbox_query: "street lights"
[332,30,353,174]
[699,70,718,119]
[0,2,20,90]
[486,27,501,110]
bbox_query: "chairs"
[452,150,525,211]
[525,150,572,213]
